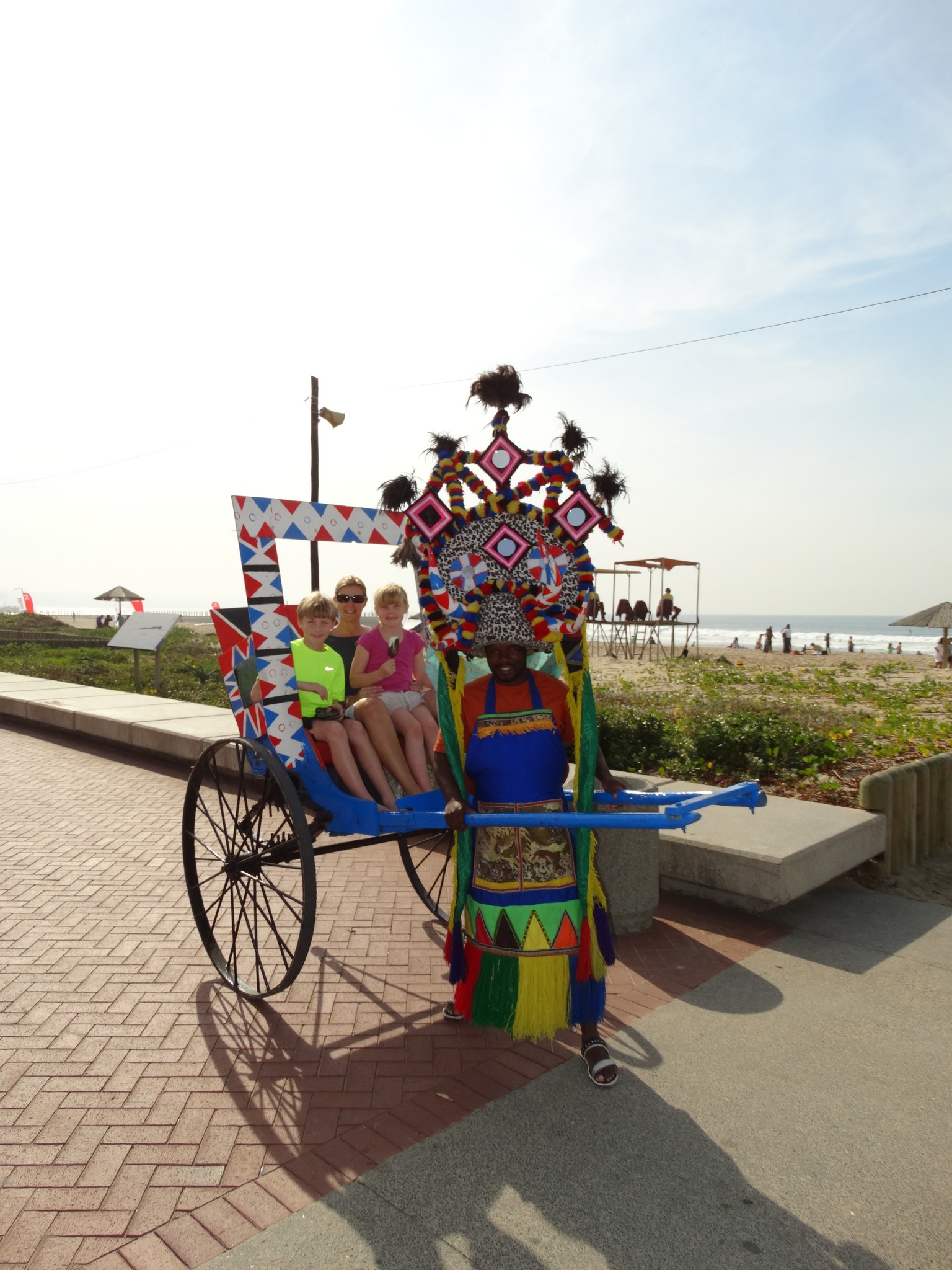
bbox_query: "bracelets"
[346,695,350,703]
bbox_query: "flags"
[130,599,143,612]
[14,588,34,613]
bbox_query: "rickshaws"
[178,365,771,1002]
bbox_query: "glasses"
[335,593,367,604]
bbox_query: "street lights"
[309,376,348,591]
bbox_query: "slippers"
[580,1037,618,1087]
[442,997,464,1019]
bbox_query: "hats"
[470,594,554,659]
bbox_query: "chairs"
[587,598,601,620]
[614,599,636,621]
[658,599,674,617]
[632,600,653,621]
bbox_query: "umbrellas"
[95,586,144,614]
[888,601,952,669]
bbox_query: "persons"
[651,637,655,644]
[588,587,605,621]
[433,593,628,1086]
[323,576,438,796]
[756,624,792,653]
[848,636,853,652]
[117,613,129,627]
[886,642,902,654]
[97,613,112,628]
[663,588,681,621]
[802,633,830,652]
[251,591,397,812]
[631,636,634,644]
[732,637,745,648]
[349,583,439,792]
[72,611,75,620]
[916,651,922,655]
[934,637,952,669]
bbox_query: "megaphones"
[319,408,345,428]
